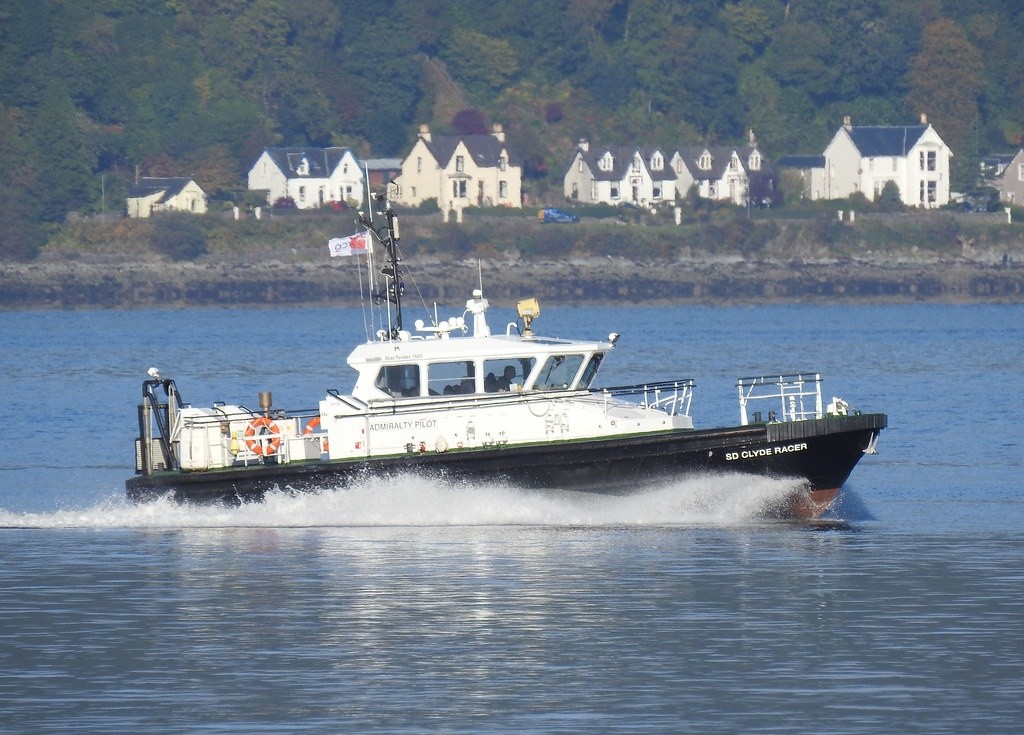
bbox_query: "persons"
[404,366,516,397]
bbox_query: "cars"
[539,208,578,224]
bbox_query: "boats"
[125,161,890,530]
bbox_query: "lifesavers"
[243,416,282,456]
[304,417,330,451]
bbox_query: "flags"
[327,231,373,258]
